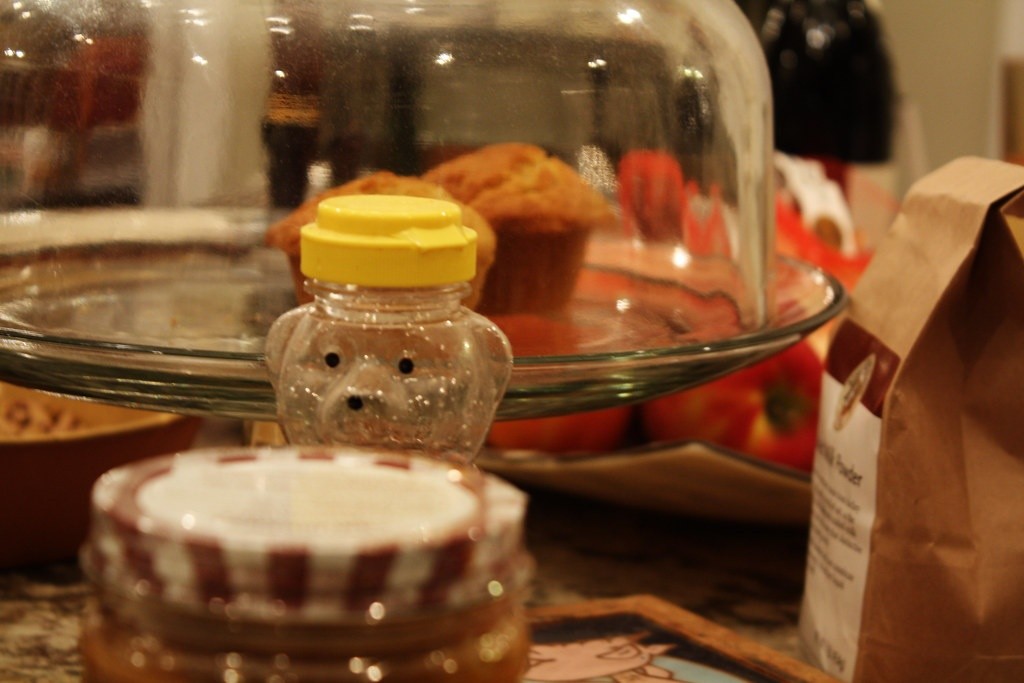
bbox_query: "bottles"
[265,194,514,465]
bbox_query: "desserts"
[260,141,609,331]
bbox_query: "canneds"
[73,441,536,683]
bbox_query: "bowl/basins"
[0,381,200,576]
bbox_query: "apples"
[477,293,822,474]
